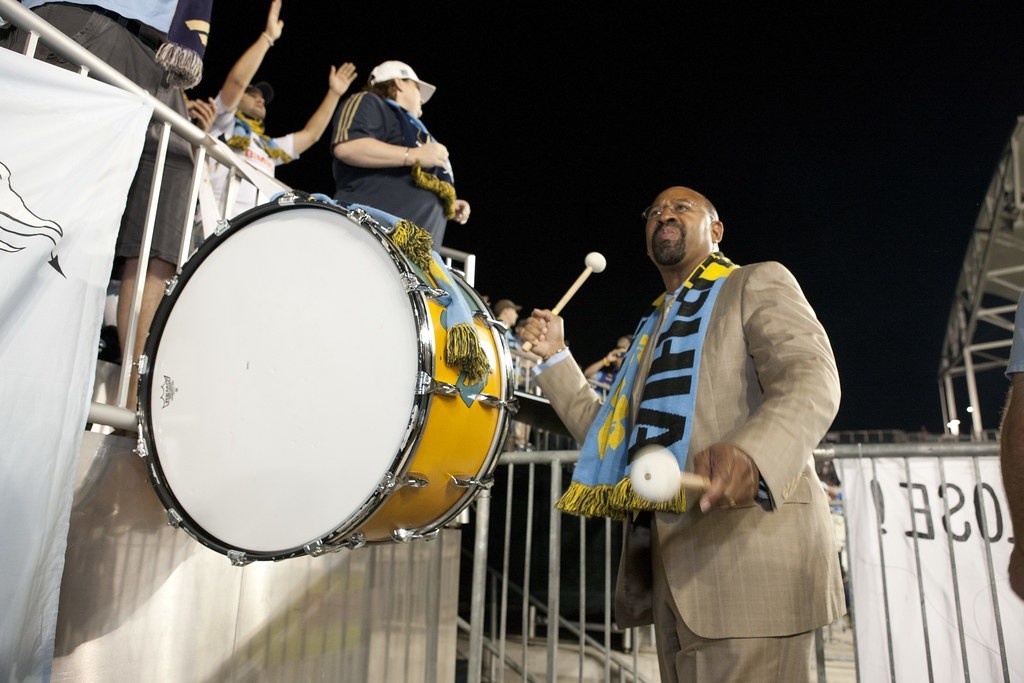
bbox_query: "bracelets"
[603,356,610,366]
[542,346,569,360]
[263,30,274,45]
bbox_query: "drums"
[133,190,520,567]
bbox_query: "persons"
[115,81,218,438]
[495,300,525,453]
[586,336,637,402]
[518,184,851,683]
[513,320,545,452]
[195,1,356,249]
[1000,278,1024,599]
[0,1,181,141]
[332,59,471,258]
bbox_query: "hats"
[245,80,274,106]
[367,60,436,106]
[494,298,522,318]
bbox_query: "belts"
[91,3,164,51]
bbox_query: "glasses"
[640,201,716,225]
